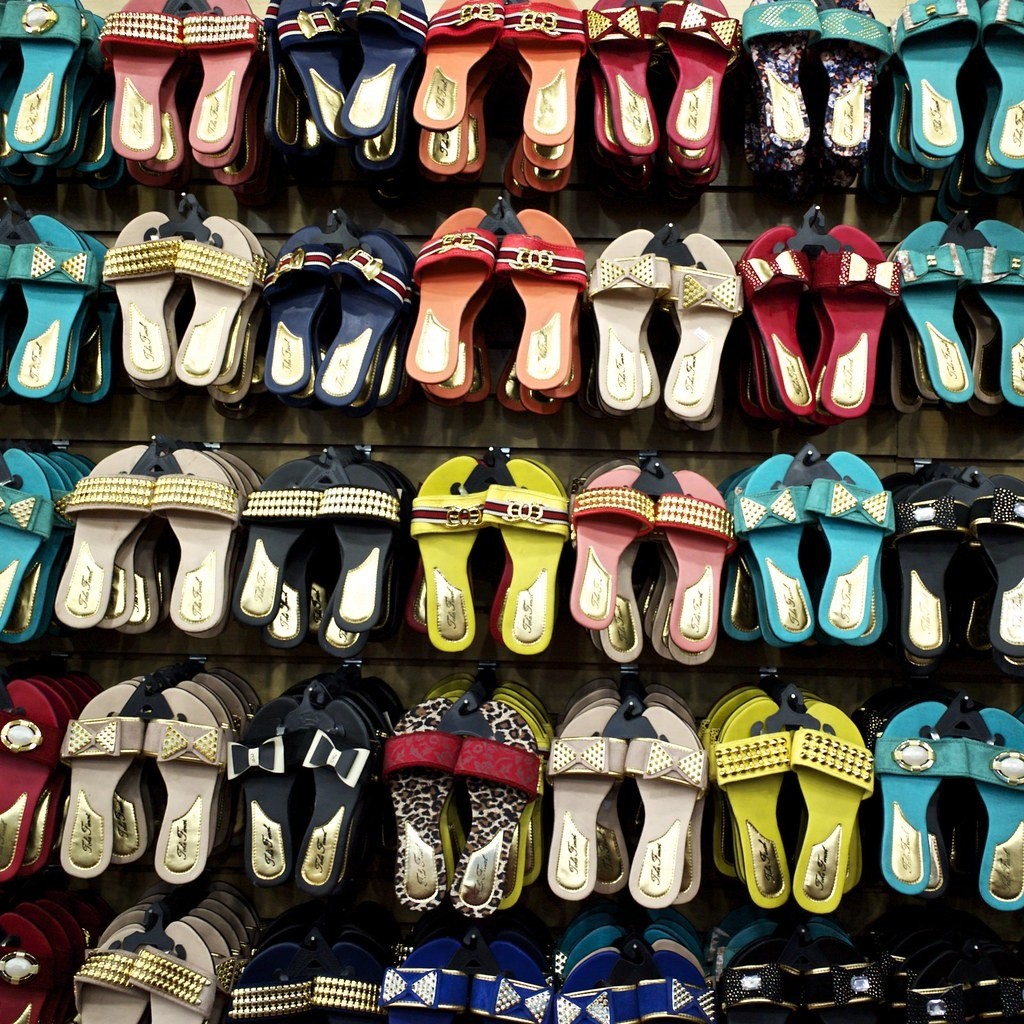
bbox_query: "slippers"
[2,0,1024,1024]
